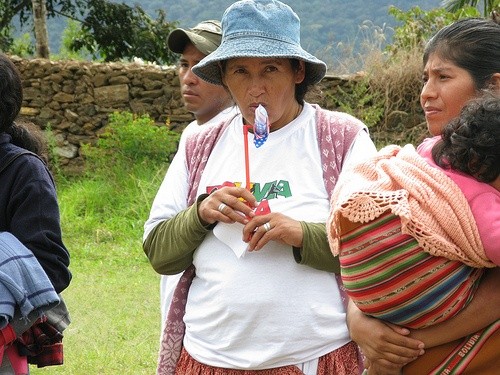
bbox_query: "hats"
[191,0,327,88]
[167,19,223,55]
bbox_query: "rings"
[217,203,226,211]
[263,223,270,232]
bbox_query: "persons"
[142,1,378,375]
[0,52,72,374]
[417,92,500,265]
[159,19,233,348]
[346,18,499,375]
